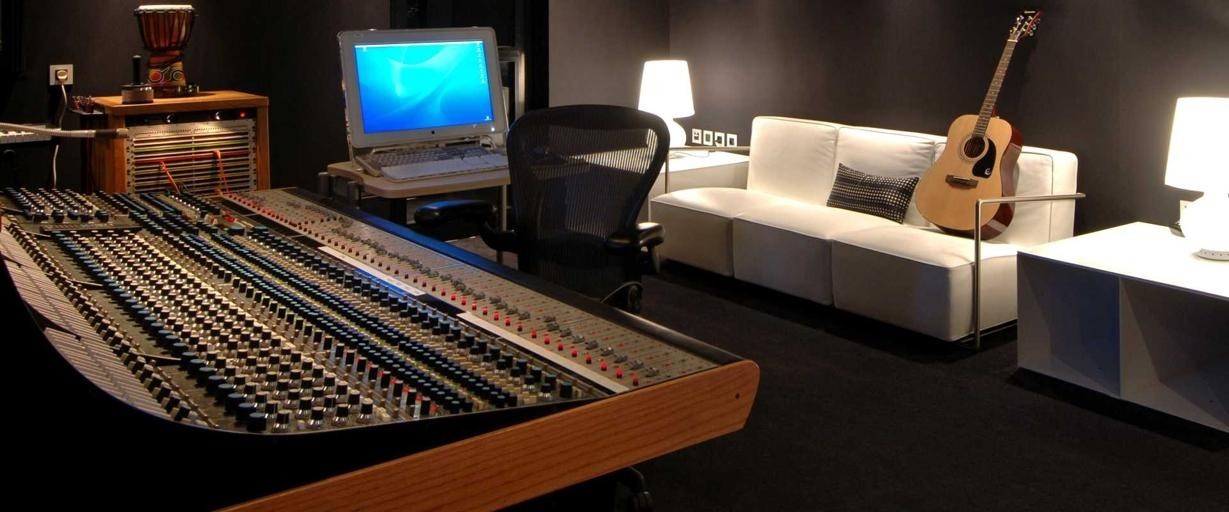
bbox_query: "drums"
[134,4,195,98]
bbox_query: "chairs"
[411,103,665,509]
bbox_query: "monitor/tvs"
[337,26,508,150]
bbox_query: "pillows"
[826,161,921,225]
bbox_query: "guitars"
[916,10,1042,239]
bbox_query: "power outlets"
[49,64,75,87]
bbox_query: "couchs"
[649,116,1078,355]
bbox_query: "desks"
[326,158,514,238]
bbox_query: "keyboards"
[355,144,510,182]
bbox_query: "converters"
[57,70,69,80]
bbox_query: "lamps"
[636,60,698,160]
[1164,96,1229,263]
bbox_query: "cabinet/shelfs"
[1014,219,1228,436]
[564,143,750,255]
[78,87,274,198]
